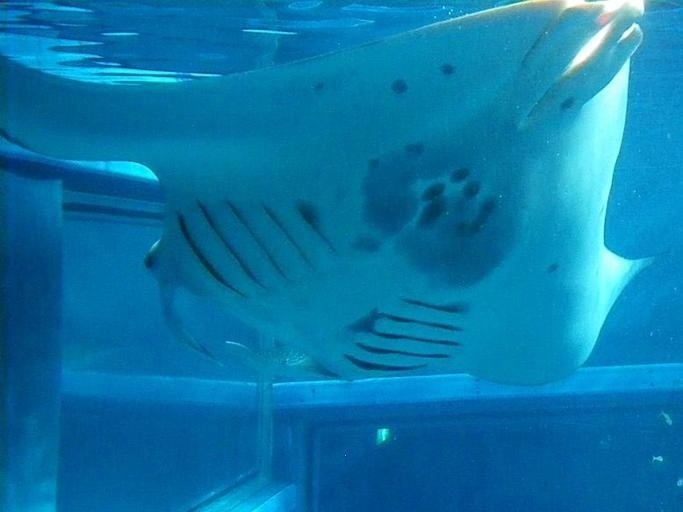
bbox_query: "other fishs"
[0,1,660,387]
